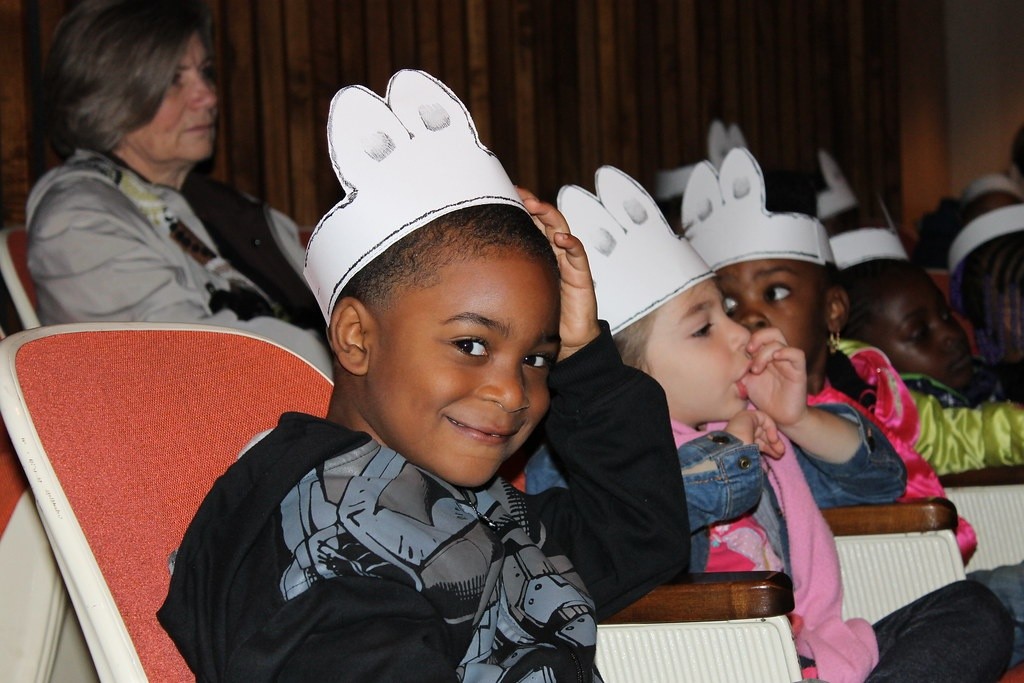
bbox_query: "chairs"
[1,212,1023,683]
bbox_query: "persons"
[553,118,1023,683]
[156,68,690,683]
[24,0,337,385]
